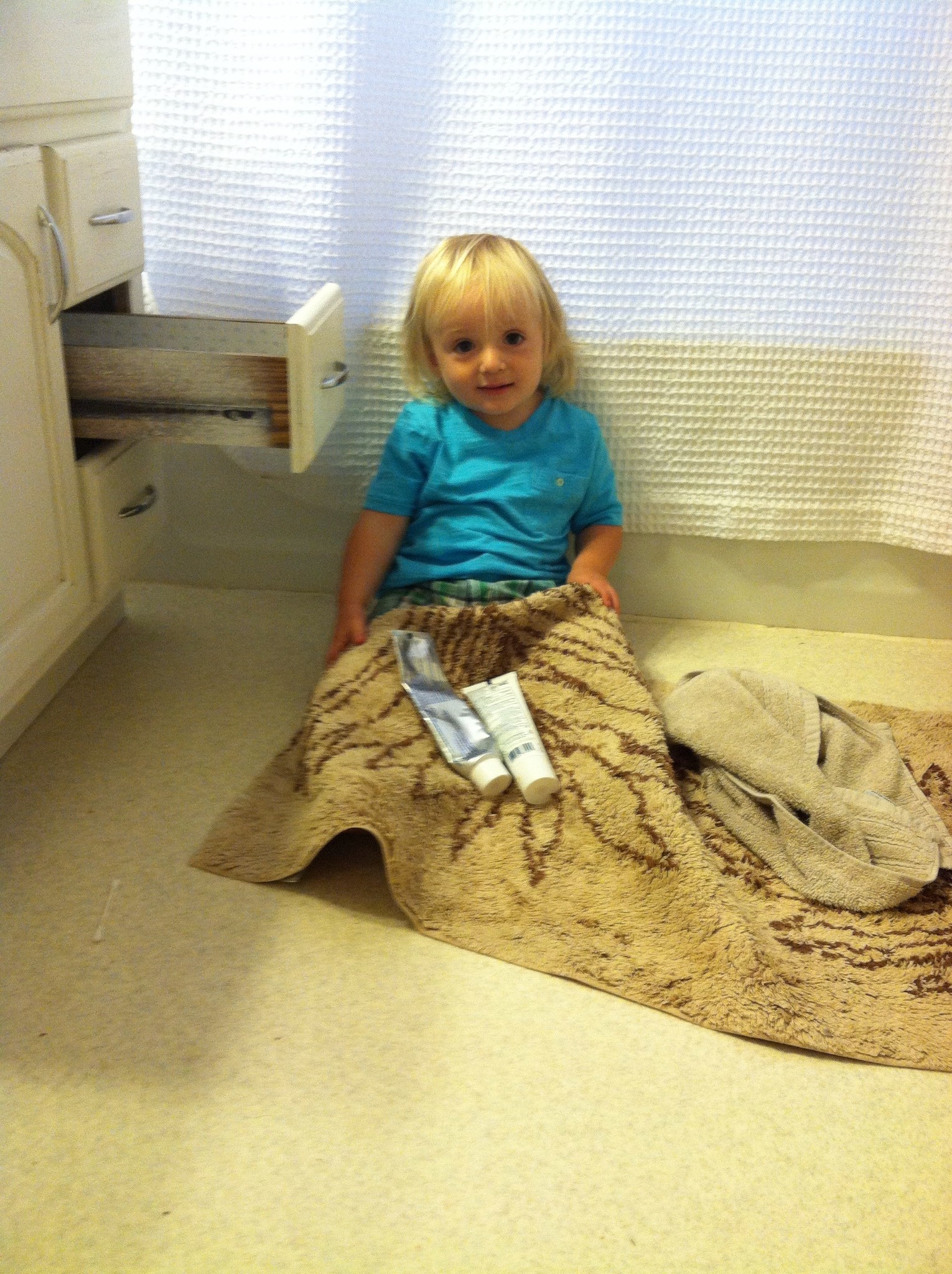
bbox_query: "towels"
[662,665,952,911]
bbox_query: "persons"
[321,234,621,666]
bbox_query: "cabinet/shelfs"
[0,1,347,761]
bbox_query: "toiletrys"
[387,625,510,798]
[461,669,559,808]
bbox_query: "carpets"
[184,581,952,1076]
